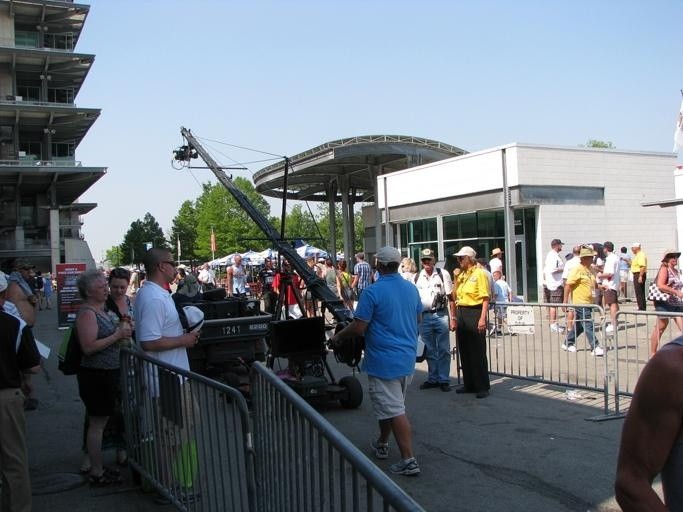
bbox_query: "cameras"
[432,292,444,310]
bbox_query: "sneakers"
[80,462,91,473]
[456,387,476,393]
[370,439,389,459]
[420,381,440,389]
[102,464,121,476]
[561,344,576,352]
[591,346,604,356]
[440,383,450,392]
[477,389,489,398]
[388,457,420,476]
[89,471,121,487]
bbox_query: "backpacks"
[58,307,98,376]
[647,265,670,302]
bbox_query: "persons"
[80,268,135,473]
[133,247,208,506]
[328,246,424,477]
[0,271,41,512]
[74,269,139,487]
[615,334,683,512]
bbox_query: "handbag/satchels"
[339,273,354,300]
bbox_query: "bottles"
[119,315,131,347]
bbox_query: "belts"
[456,306,480,310]
[422,307,447,315]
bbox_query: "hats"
[491,247,504,257]
[0,270,8,293]
[420,249,435,260]
[452,245,477,258]
[373,245,402,266]
[551,239,564,246]
[176,304,204,333]
[14,257,35,269]
[578,248,597,258]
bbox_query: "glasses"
[162,260,178,267]
